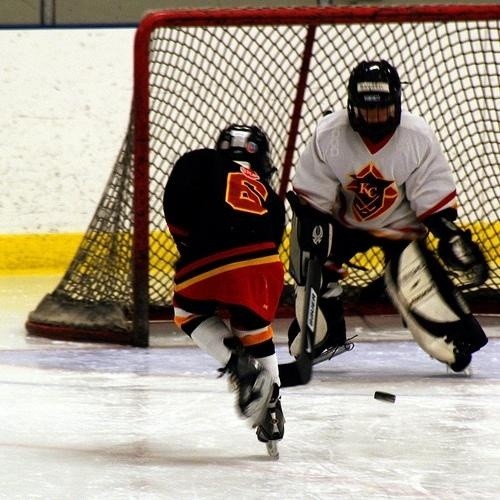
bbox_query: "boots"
[216,335,273,418]
[256,382,284,443]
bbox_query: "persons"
[161,120,287,460]
[287,58,489,378]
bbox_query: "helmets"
[347,59,402,140]
[215,123,270,162]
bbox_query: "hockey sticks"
[278,255,325,387]
